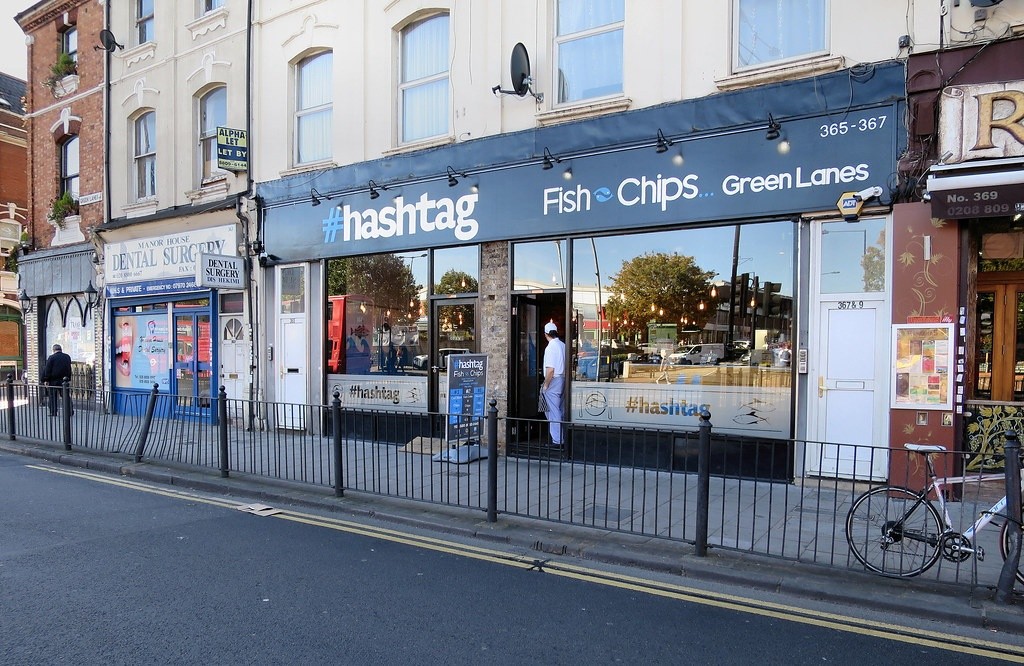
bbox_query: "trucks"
[668,343,725,366]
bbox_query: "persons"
[655,353,674,384]
[116,315,137,388]
[41,344,74,417]
[773,342,792,367]
[540,323,565,450]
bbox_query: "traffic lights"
[762,281,782,317]
[729,273,749,318]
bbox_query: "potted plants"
[41,53,76,101]
[47,191,78,229]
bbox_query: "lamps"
[766,114,781,140]
[446,166,467,187]
[18,289,34,324]
[368,180,386,199]
[84,280,101,318]
[656,129,673,154]
[311,188,330,207]
[541,146,560,169]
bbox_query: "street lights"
[710,284,720,343]
[399,254,427,326]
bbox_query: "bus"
[285,294,377,377]
[734,340,751,349]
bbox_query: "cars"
[648,354,661,363]
[576,356,619,378]
[739,353,751,364]
[638,343,659,354]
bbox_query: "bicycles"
[845,443,1024,585]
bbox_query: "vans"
[604,339,623,350]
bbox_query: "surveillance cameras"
[853,187,877,202]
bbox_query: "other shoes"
[546,443,564,451]
[48,413,57,417]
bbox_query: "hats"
[545,323,557,333]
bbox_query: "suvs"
[414,347,474,371]
